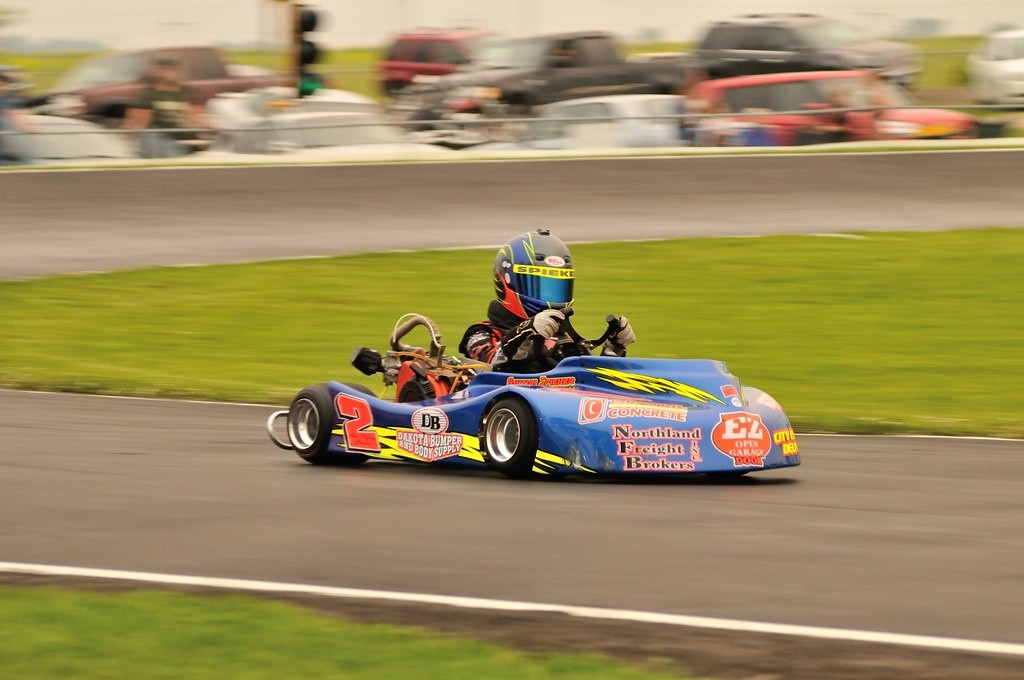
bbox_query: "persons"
[461,229,635,376]
[144,56,201,151]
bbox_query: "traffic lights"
[291,4,318,98]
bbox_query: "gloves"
[601,316,636,358]
[501,309,565,359]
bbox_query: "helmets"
[493,228,575,318]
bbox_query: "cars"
[0,6,985,165]
[964,28,1024,109]
